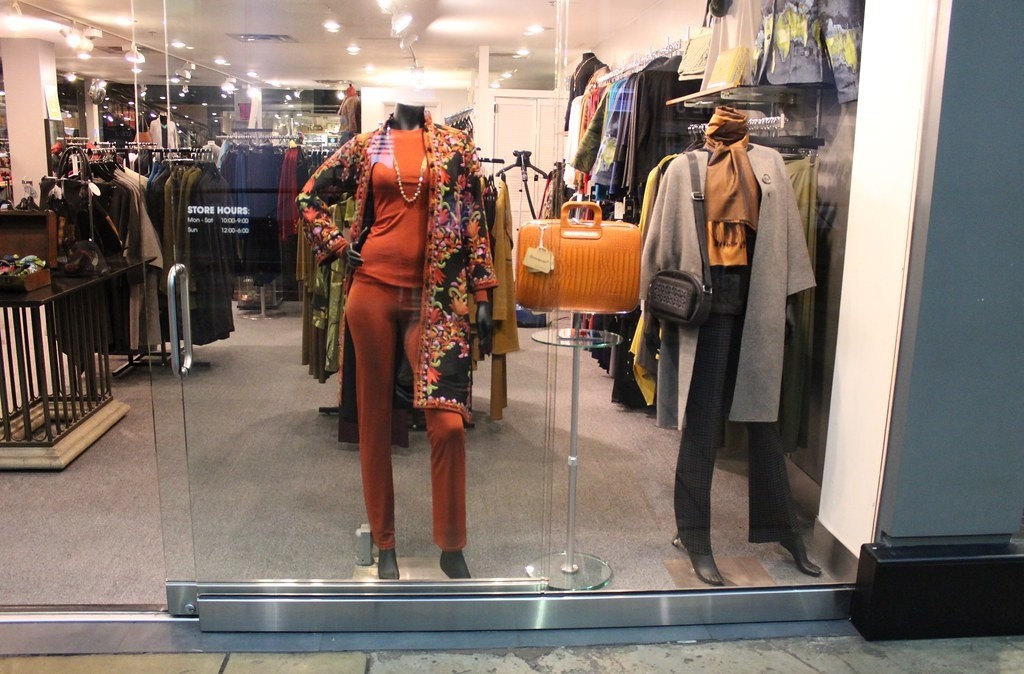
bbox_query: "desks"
[0,255,157,470]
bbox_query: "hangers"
[594,40,682,90]
[304,142,339,178]
[444,108,476,134]
[65,135,219,180]
[680,118,809,161]
[477,156,505,200]
[224,134,301,149]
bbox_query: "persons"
[640,107,824,586]
[338,82,361,143]
[150,111,179,149]
[294,99,498,578]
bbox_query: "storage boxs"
[0,269,52,293]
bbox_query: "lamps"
[346,42,362,53]
[285,94,293,100]
[294,89,304,98]
[392,13,413,35]
[322,18,342,30]
[182,86,189,93]
[128,99,135,104]
[176,68,193,79]
[201,100,208,105]
[171,73,181,84]
[105,95,110,101]
[275,112,281,120]
[220,82,235,91]
[125,49,146,64]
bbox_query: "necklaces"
[386,123,428,202]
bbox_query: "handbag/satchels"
[641,151,714,328]
[39,146,114,225]
[133,118,154,145]
[515,200,644,315]
[51,237,113,278]
